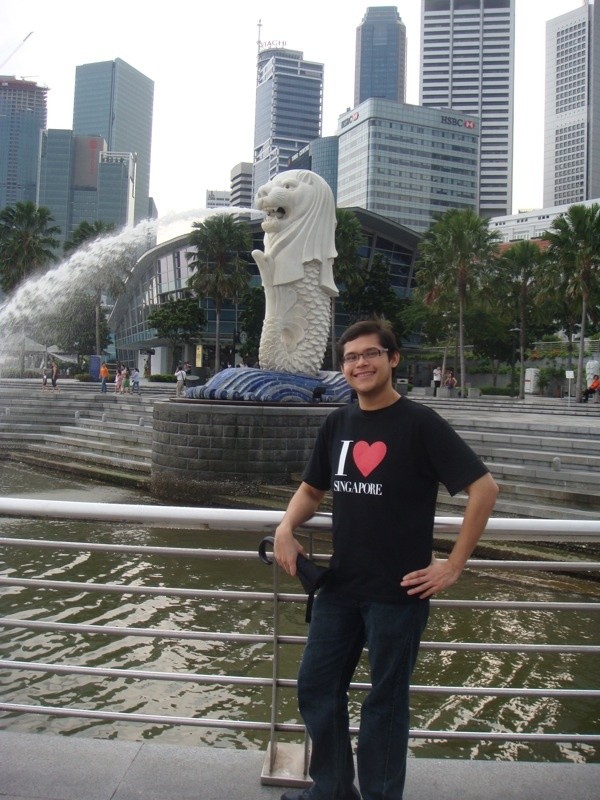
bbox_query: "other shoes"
[281,789,315,800]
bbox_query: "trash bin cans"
[395,379,408,395]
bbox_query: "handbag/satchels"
[184,380,186,385]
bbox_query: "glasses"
[343,347,389,364]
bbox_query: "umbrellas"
[260,537,330,622]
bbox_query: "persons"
[175,367,186,396]
[239,359,259,368]
[581,375,600,403]
[184,361,192,374]
[67,363,86,378]
[99,362,108,393]
[113,365,141,395]
[433,367,441,396]
[42,361,60,392]
[273,321,500,800]
[444,373,457,395]
